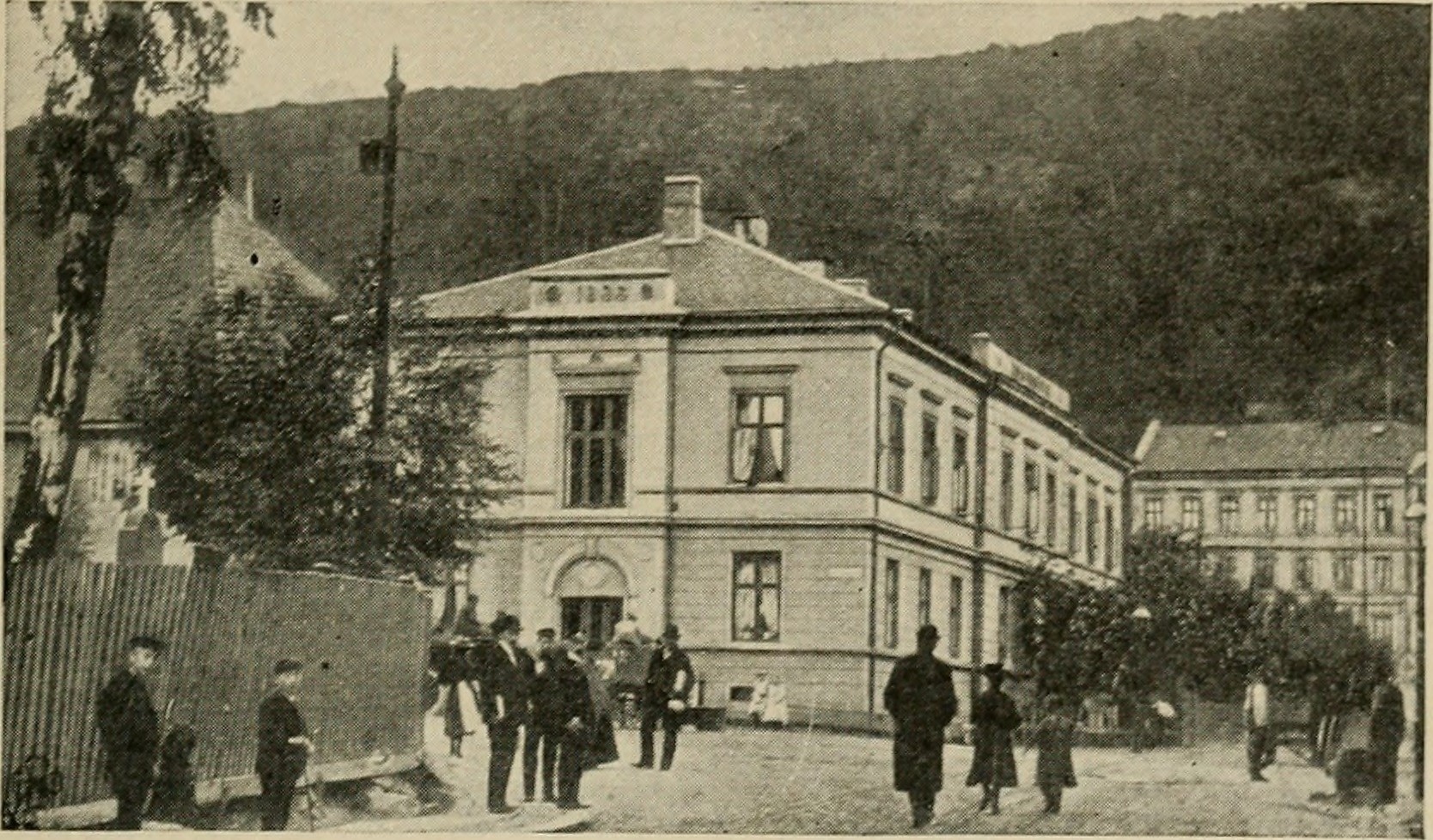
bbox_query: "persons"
[97,636,166,830]
[750,672,788,729]
[615,616,642,649]
[442,619,614,814]
[456,598,483,659]
[965,665,1019,815]
[1025,697,1076,812]
[1243,672,1274,781]
[256,659,315,830]
[883,626,956,826]
[1368,672,1402,804]
[630,624,695,771]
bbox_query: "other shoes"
[632,761,674,772]
[487,795,590,814]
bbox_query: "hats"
[493,615,521,631]
[984,663,1004,676]
[916,624,941,640]
[660,622,682,639]
[126,636,168,655]
